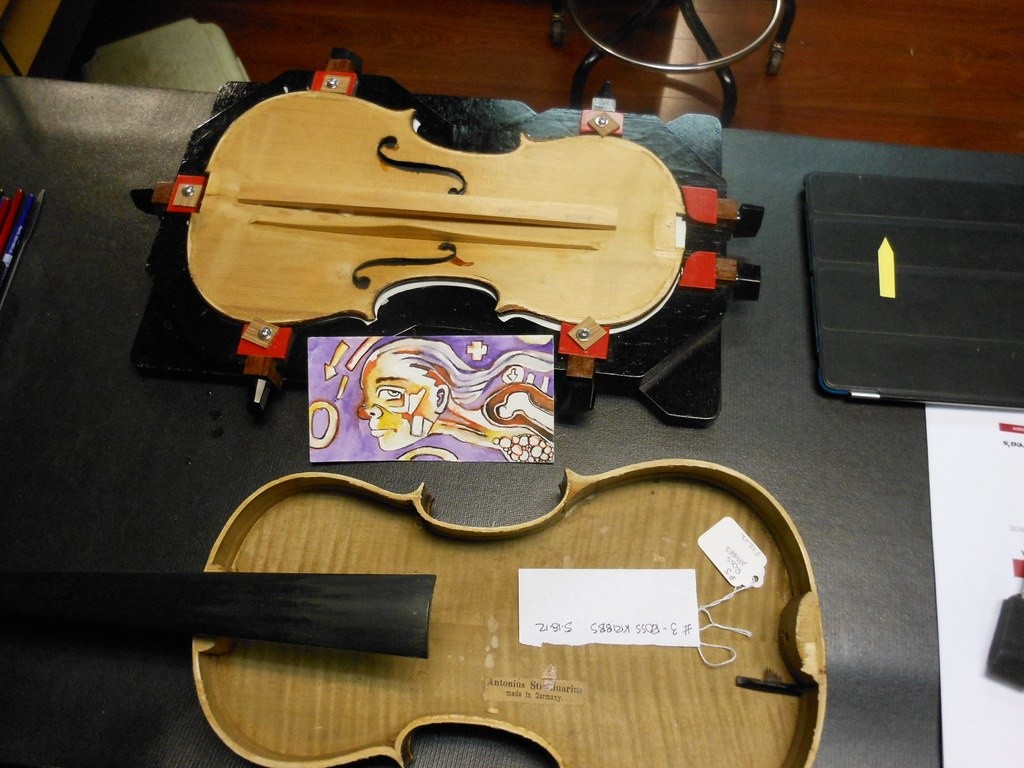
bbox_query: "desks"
[0,74,1024,768]
